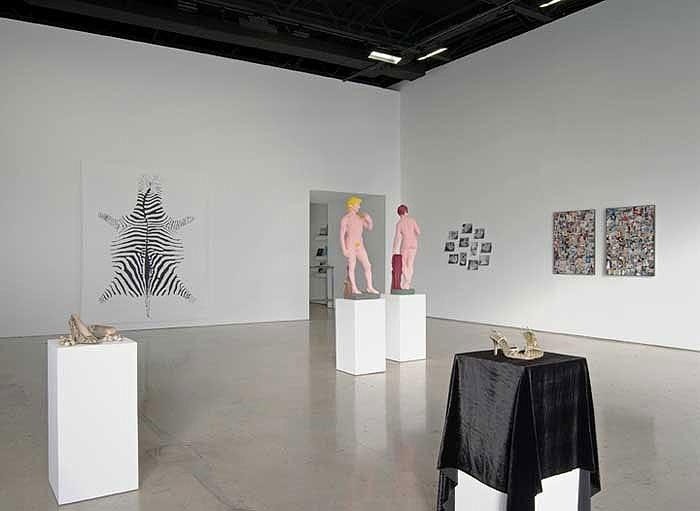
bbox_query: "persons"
[339,195,379,294]
[445,224,493,269]
[391,204,421,288]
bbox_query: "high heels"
[69,314,116,344]
[490,324,544,360]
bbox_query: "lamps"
[416,41,448,60]
[365,44,402,67]
[537,0,561,8]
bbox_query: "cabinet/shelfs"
[315,235,328,278]
